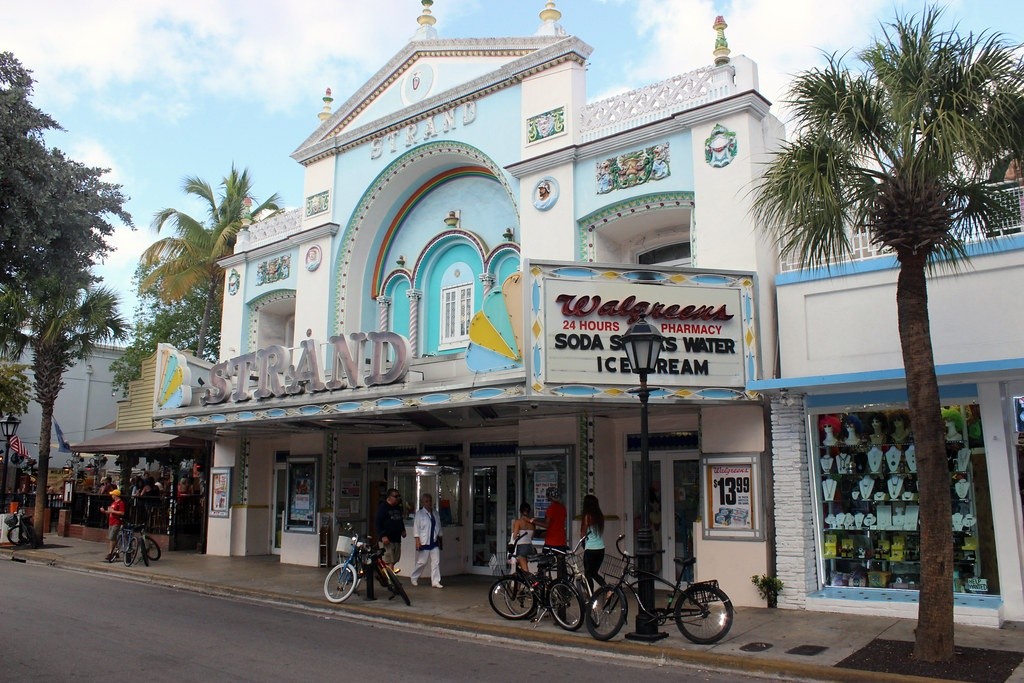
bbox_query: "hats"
[109,490,121,496]
[547,487,560,501]
[100,477,107,483]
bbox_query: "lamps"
[198,377,205,388]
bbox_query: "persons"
[820,409,964,446]
[581,495,613,608]
[513,503,537,589]
[410,494,443,589]
[375,488,406,576]
[523,486,570,602]
[99,472,204,498]
[101,489,125,559]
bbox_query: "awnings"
[69,429,204,452]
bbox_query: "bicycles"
[5,511,37,549]
[109,518,138,566]
[487,532,600,631]
[134,520,161,566]
[323,522,365,603]
[364,537,411,606]
[584,535,737,646]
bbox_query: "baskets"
[4,515,18,527]
[599,554,635,581]
[336,536,354,556]
[488,552,511,577]
[564,550,586,573]
[687,580,720,605]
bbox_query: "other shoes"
[412,581,418,586]
[603,591,614,610]
[432,584,443,588]
[105,552,119,559]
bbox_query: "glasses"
[872,420,880,423]
[893,418,902,421]
[824,424,831,428]
[845,423,854,428]
[392,495,401,498]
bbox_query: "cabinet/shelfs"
[816,440,978,592]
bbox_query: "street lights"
[616,311,668,640]
[0,411,22,514]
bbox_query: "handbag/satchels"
[438,536,443,550]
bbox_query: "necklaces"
[946,431,958,439]
[873,432,882,438]
[821,450,971,530]
[894,430,906,439]
[846,436,859,443]
